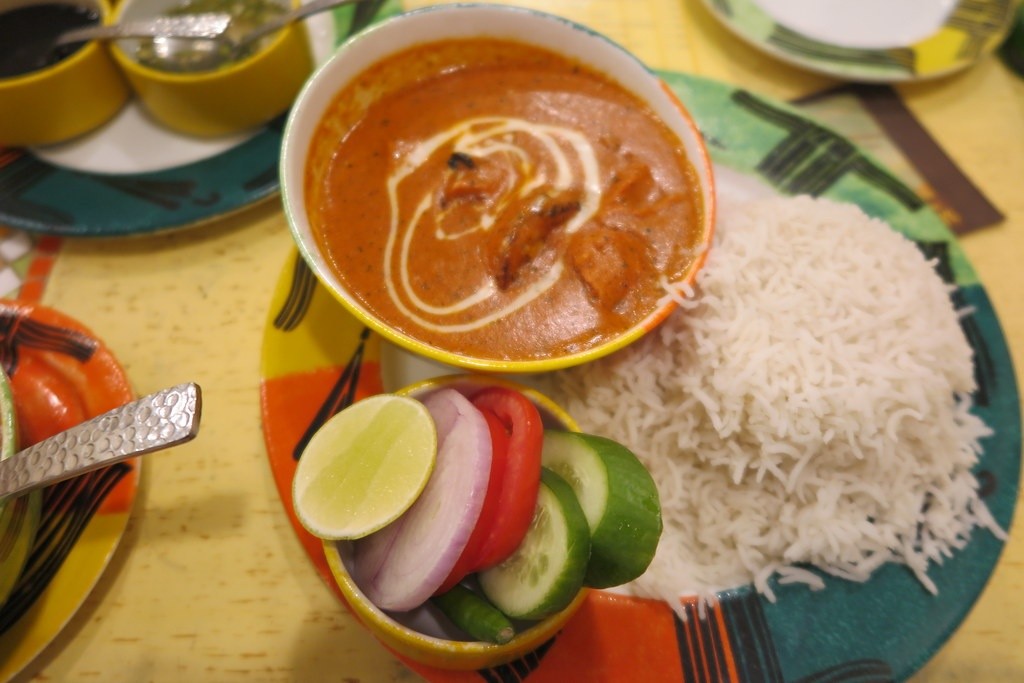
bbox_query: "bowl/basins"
[277,1,718,375]
[321,374,594,672]
[107,0,320,141]
[0,0,131,149]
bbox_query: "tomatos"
[437,387,543,593]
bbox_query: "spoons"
[152,0,383,74]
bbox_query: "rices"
[553,172,1007,622]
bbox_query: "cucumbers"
[476,469,593,619]
[538,429,662,587]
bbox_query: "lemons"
[291,394,437,541]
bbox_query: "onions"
[357,389,491,610]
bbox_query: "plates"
[0,299,145,683]
[258,67,1024,683]
[701,1,1024,85]
[0,0,410,238]
